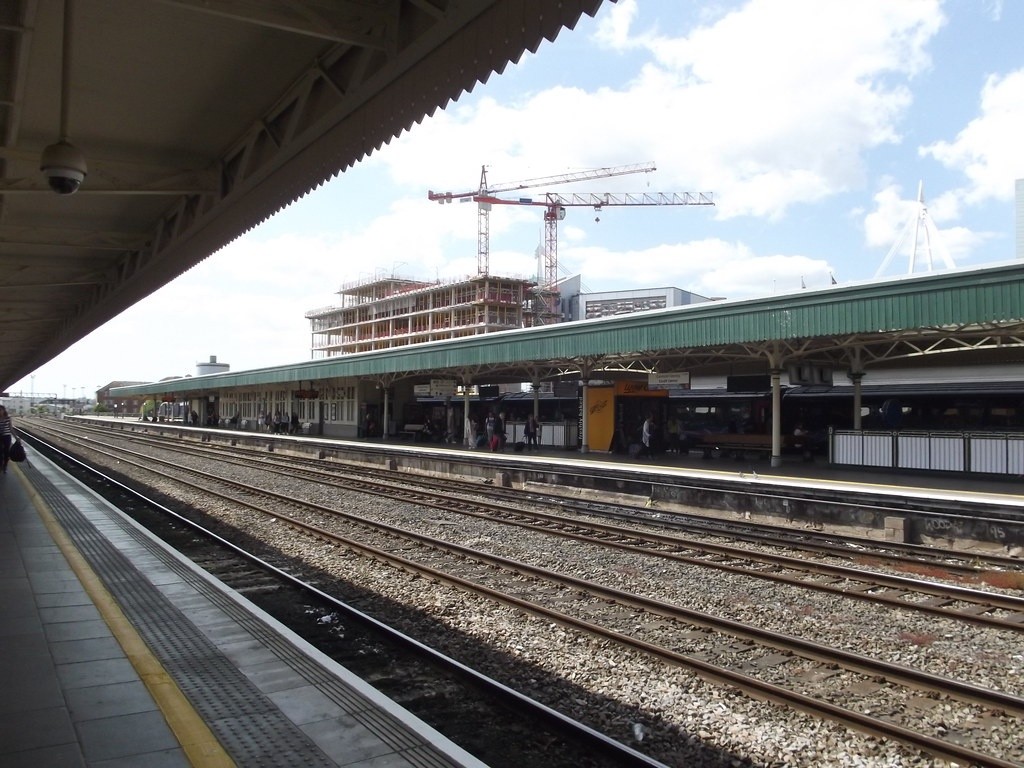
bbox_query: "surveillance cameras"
[39,141,88,194]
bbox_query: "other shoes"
[445,438,448,443]
[451,441,456,444]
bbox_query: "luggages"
[679,433,689,454]
[628,437,649,458]
[514,436,527,452]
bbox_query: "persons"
[790,421,815,462]
[0,405,19,473]
[191,410,198,423]
[485,411,506,452]
[465,415,479,450]
[265,411,299,435]
[445,409,457,444]
[421,419,435,442]
[634,411,657,458]
[666,411,682,454]
[524,414,539,451]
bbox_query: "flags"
[801,276,806,288]
[831,274,837,284]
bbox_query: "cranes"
[424,159,718,326]
